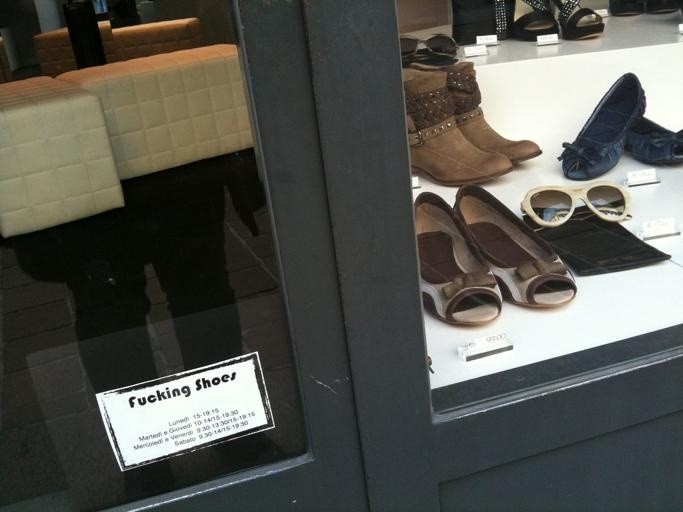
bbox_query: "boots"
[401,48,542,187]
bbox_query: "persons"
[14,148,288,503]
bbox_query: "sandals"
[511,0,605,42]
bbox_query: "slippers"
[414,183,577,325]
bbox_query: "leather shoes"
[557,72,682,180]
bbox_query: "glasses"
[520,180,631,228]
[400,33,457,57]
